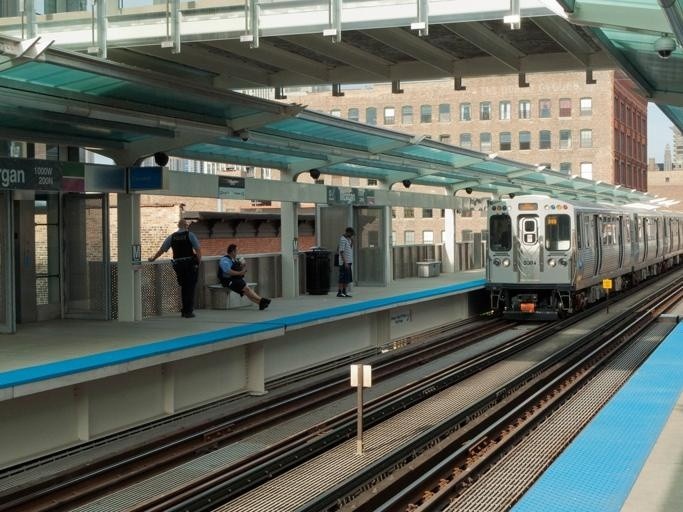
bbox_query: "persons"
[218,245,271,310]
[337,228,354,297]
[148,219,201,318]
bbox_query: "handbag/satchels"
[333,253,339,266]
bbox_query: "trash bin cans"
[303,247,332,295]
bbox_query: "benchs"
[208,282,260,309]
[416,260,442,279]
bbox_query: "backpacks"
[231,260,245,278]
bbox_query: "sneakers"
[181,310,195,318]
[258,297,271,311]
[336,292,352,297]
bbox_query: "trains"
[487,194,683,320]
